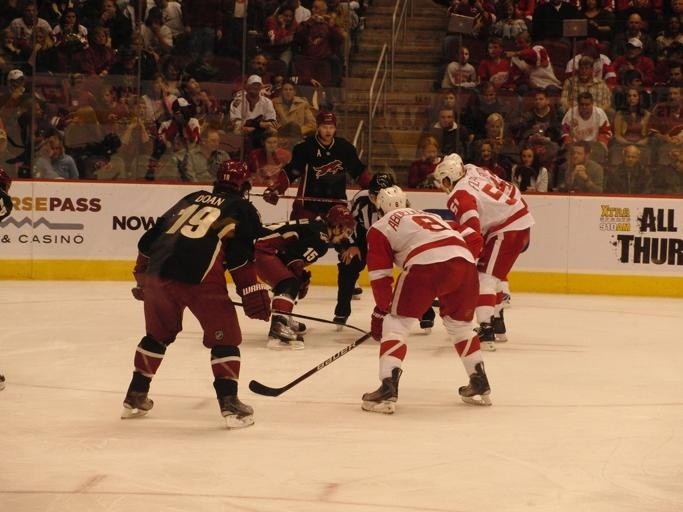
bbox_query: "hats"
[7,69,25,81]
[246,74,265,88]
[172,97,194,112]
[624,36,643,49]
[581,37,607,50]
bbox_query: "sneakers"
[334,314,348,324]
[420,307,435,329]
[353,280,362,294]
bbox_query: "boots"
[362,367,403,402]
[493,308,506,333]
[288,315,307,335]
[473,315,495,342]
[458,361,490,397]
[123,372,154,411]
[213,380,254,417]
[269,314,304,342]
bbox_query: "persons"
[0,127,11,389]
[334,172,436,328]
[123,162,270,416]
[434,153,536,342]
[262,111,373,296]
[362,186,491,402]
[254,204,354,341]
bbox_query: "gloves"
[243,282,272,321]
[290,269,312,285]
[263,184,281,205]
[132,271,146,301]
[371,307,386,342]
[298,283,310,300]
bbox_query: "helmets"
[367,172,395,204]
[213,159,254,197]
[375,184,407,219]
[432,151,465,195]
[316,112,337,128]
[325,204,355,245]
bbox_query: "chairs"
[408,112,681,188]
[3,1,364,88]
[3,95,319,182]
[438,3,680,109]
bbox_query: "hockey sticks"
[233,301,371,335]
[249,332,373,398]
[243,190,353,204]
